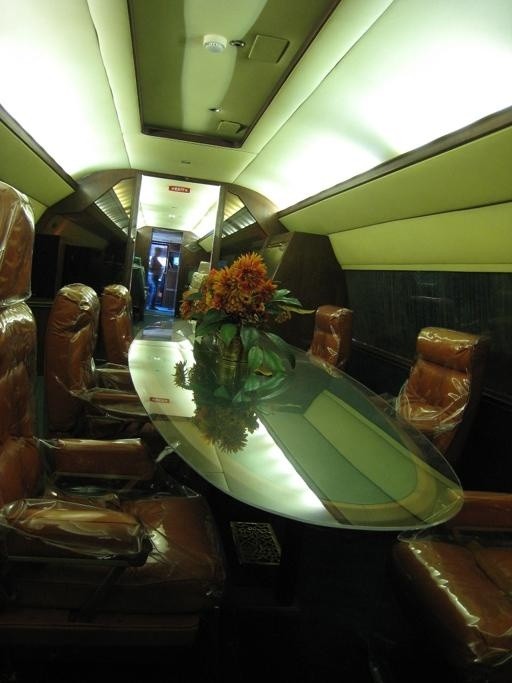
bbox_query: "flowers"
[180,250,316,377]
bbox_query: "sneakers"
[146,304,159,311]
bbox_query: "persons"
[149,247,162,310]
